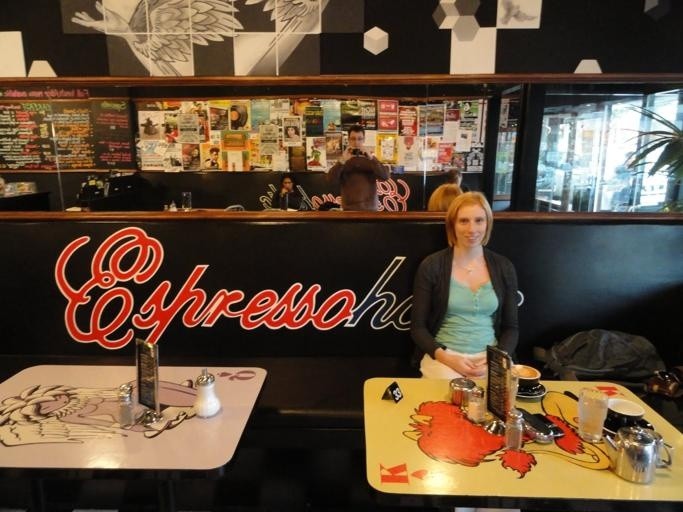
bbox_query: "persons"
[284,126,299,139]
[326,124,390,211]
[270,175,301,208]
[408,189,520,380]
[204,148,219,167]
[444,168,463,186]
[427,184,463,212]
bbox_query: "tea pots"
[605,424,670,484]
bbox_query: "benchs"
[0,171,486,212]
[0,221,682,419]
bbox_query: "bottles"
[504,411,525,450]
[468,388,486,423]
[118,384,134,428]
[194,368,221,418]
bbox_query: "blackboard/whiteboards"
[0,97,141,173]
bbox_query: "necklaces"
[453,260,485,274]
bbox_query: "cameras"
[351,149,360,154]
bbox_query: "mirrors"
[0,74,683,225]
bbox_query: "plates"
[516,385,547,399]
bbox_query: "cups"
[183,192,192,209]
[512,365,541,389]
[577,387,654,443]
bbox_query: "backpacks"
[541,328,665,394]
